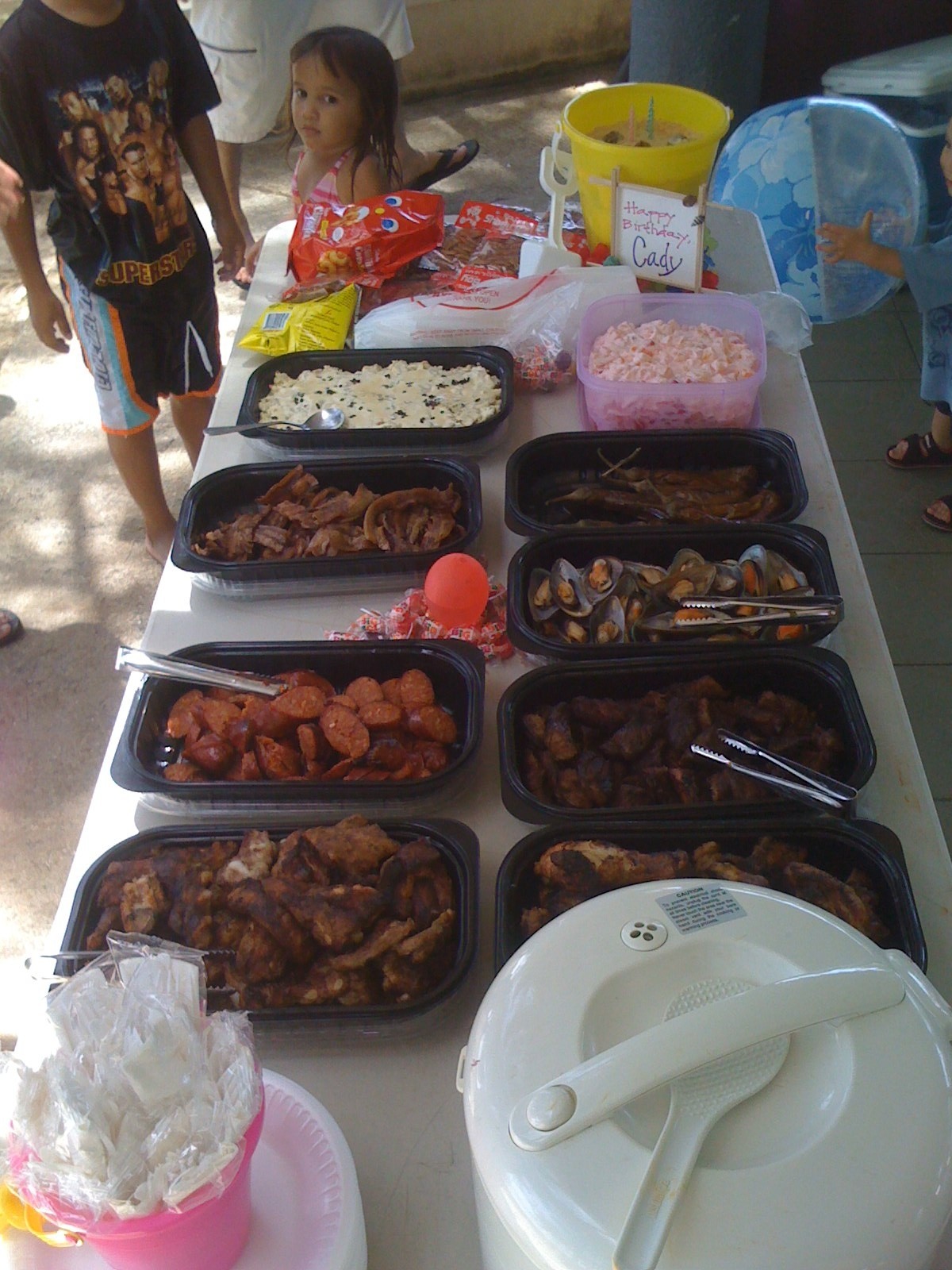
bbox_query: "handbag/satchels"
[354,264,640,347]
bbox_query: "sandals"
[885,430,952,469]
[0,608,22,647]
[924,495,952,531]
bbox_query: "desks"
[0,205,952,1270]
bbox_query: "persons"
[0,608,24,645]
[0,0,246,566]
[813,117,952,532]
[183,0,480,291]
[244,26,403,279]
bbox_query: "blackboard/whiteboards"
[610,181,706,292]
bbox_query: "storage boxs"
[571,288,771,426]
[47,813,481,1043]
[108,637,487,816]
[496,644,879,831]
[238,344,517,459]
[503,425,813,541]
[492,820,929,981]
[504,522,847,666]
[168,460,485,604]
[820,33,952,211]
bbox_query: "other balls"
[706,97,926,325]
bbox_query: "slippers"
[407,139,479,192]
[233,259,256,290]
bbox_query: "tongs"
[690,729,858,820]
[114,647,289,696]
[24,951,239,992]
[675,595,845,629]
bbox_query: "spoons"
[202,409,345,436]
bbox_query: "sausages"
[160,671,456,781]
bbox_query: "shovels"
[612,977,791,1270]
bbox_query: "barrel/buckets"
[552,82,735,253]
[0,1016,265,1270]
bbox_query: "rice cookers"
[455,878,951,1270]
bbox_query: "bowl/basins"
[496,430,928,979]
[47,346,512,1021]
[576,293,768,431]
